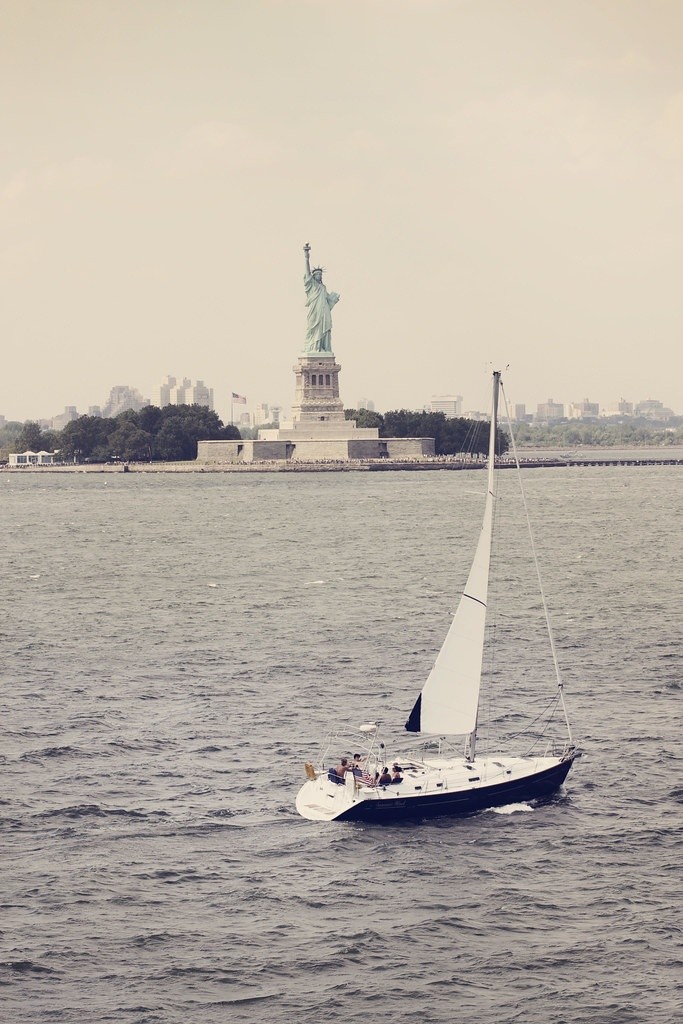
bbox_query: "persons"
[379,766,403,784]
[302,243,341,351]
[337,754,362,777]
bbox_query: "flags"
[232,392,246,404]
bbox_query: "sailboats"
[293,366,583,821]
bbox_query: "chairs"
[327,768,338,785]
[391,777,403,785]
